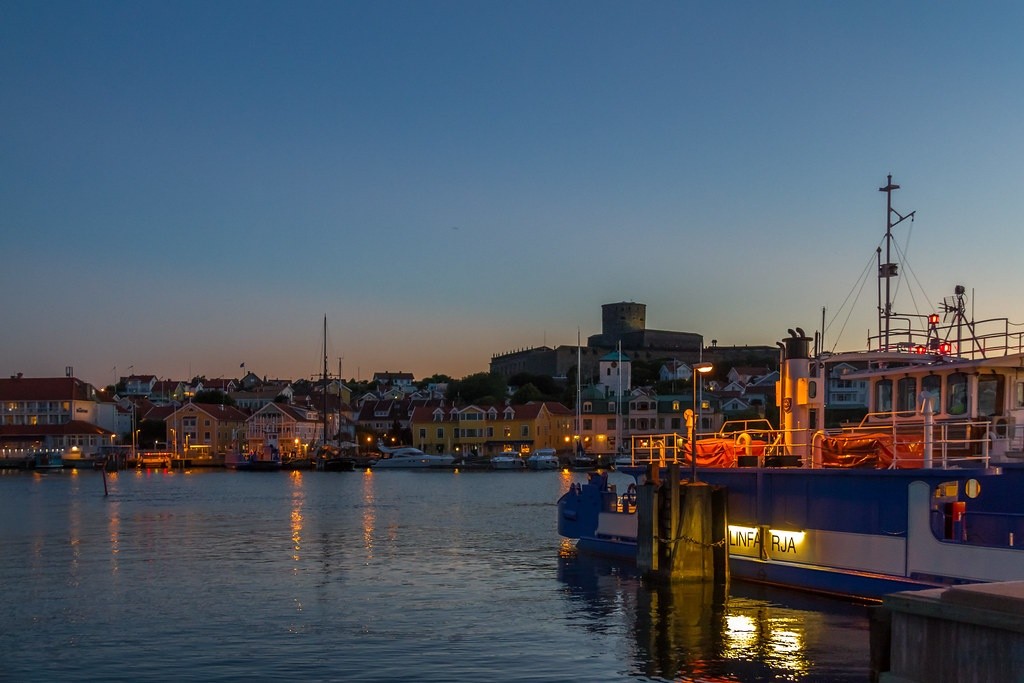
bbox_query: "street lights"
[136,429,141,449]
[110,434,116,454]
[185,435,191,456]
[692,362,713,483]
[171,429,177,456]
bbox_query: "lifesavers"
[627,483,638,506]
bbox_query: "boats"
[489,451,525,470]
[555,171,1024,603]
[315,441,359,472]
[525,448,559,470]
[571,456,595,467]
[369,444,458,468]
